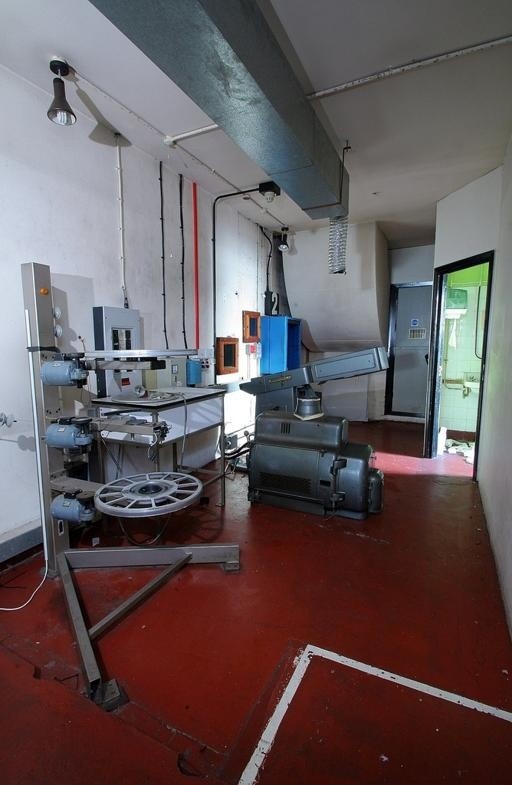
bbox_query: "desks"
[88,385,232,523]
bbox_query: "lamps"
[278,233,290,253]
[48,78,78,126]
